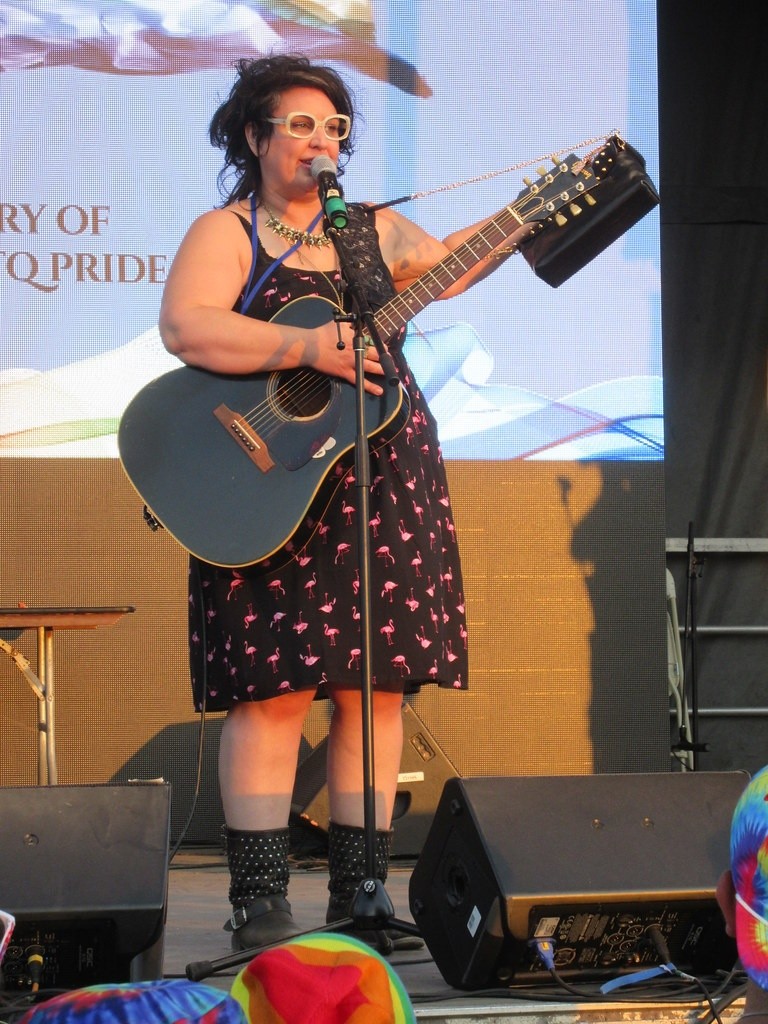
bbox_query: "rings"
[363,347,369,359]
[363,335,375,346]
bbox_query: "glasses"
[266,111,351,141]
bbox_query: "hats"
[729,764,768,997]
[230,932,420,1024]
[17,980,250,1023]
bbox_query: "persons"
[158,52,539,955]
[717,764,768,1024]
[15,931,418,1024]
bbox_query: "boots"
[220,823,305,957]
[325,819,425,951]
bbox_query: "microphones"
[312,155,349,229]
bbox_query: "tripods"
[184,215,424,984]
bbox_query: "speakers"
[0,783,172,1012]
[408,768,751,992]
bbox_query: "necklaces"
[740,1010,768,1019]
[241,191,344,316]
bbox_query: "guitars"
[115,145,606,585]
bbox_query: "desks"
[0,604,136,789]
